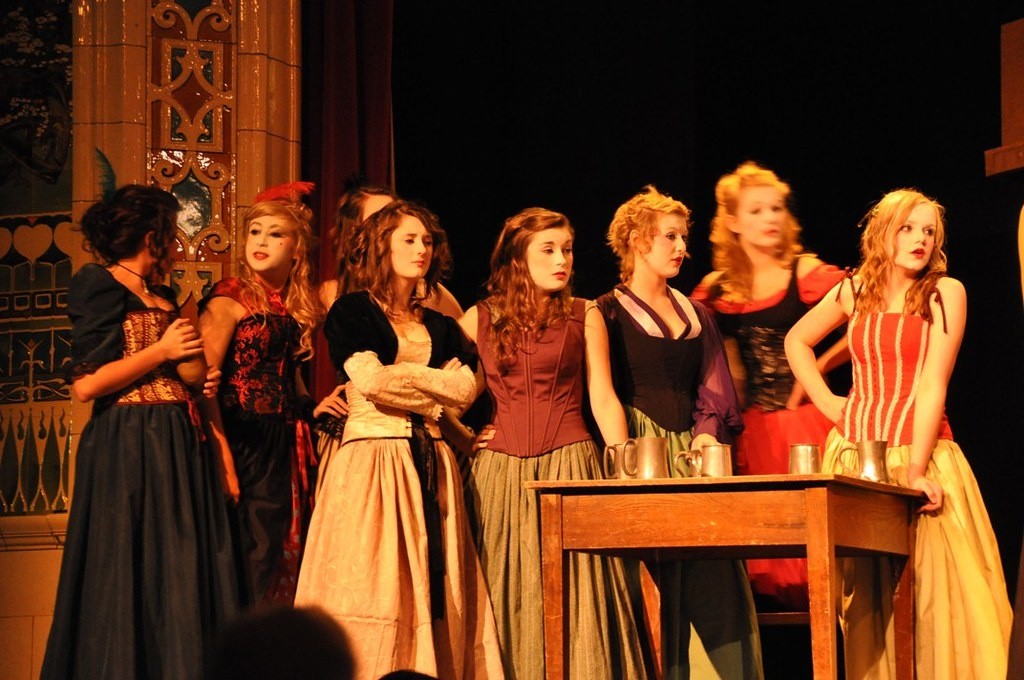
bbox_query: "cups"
[789,445,822,475]
[604,443,636,480]
[622,437,669,479]
[693,445,732,477]
[838,441,890,483]
[674,450,702,477]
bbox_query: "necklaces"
[115,260,150,293]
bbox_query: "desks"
[523,473,929,679]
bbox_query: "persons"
[37,185,245,680]
[190,161,1013,680]
